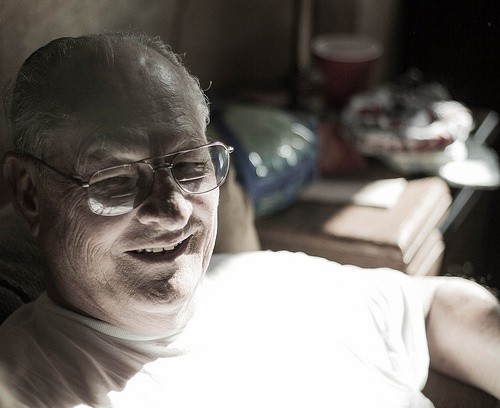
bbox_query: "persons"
[0,29,500,408]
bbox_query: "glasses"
[16,137,234,216]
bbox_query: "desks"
[255,108,500,276]
